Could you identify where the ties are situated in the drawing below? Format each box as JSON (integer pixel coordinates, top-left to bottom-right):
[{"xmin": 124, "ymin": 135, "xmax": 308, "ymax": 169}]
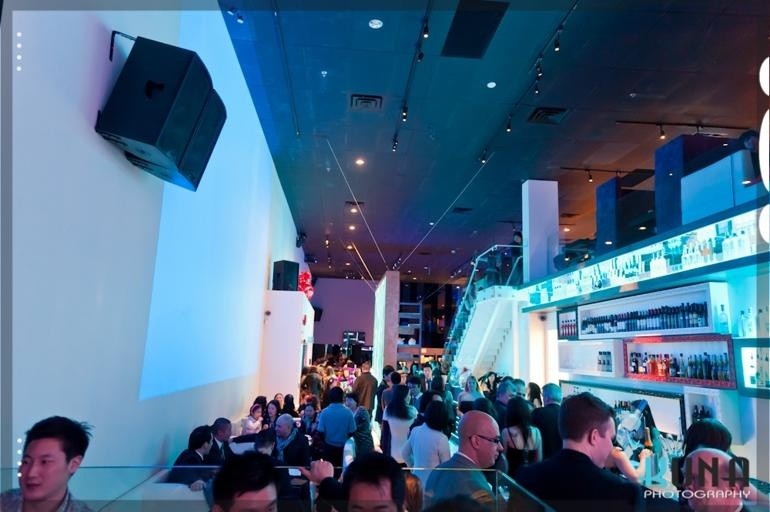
[{"xmin": 220, "ymin": 445, "xmax": 226, "ymax": 459}]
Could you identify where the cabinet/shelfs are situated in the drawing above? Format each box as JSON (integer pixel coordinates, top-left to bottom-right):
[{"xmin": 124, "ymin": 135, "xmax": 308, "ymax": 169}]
[{"xmin": 556, "ymin": 281, "xmax": 731, "ymax": 442}]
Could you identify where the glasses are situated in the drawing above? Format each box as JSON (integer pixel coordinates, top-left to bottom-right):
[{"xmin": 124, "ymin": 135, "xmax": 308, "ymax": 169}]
[{"xmin": 476, "ymin": 434, "xmax": 501, "ymax": 444}]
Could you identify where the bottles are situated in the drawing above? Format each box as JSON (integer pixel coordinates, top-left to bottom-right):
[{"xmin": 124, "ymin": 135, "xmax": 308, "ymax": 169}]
[
  {"xmin": 573, "ymin": 386, "xmax": 592, "ymax": 397},
  {"xmin": 692, "ymin": 404, "xmax": 710, "ymax": 423},
  {"xmin": 580, "ymin": 300, "xmax": 708, "ymax": 335},
  {"xmin": 614, "ymin": 399, "xmax": 631, "ymax": 424},
  {"xmin": 750, "ymin": 354, "xmax": 769, "ymax": 387},
  {"xmin": 629, "ymin": 352, "xmax": 730, "ymax": 382},
  {"xmin": 553, "ymin": 219, "xmax": 752, "ymax": 297},
  {"xmin": 560, "ymin": 318, "xmax": 576, "ymax": 336},
  {"xmin": 596, "ymin": 351, "xmax": 612, "ymax": 373},
  {"xmin": 644, "ymin": 427, "xmax": 654, "ymax": 454},
  {"xmin": 719, "ymin": 304, "xmax": 769, "ymax": 338}
]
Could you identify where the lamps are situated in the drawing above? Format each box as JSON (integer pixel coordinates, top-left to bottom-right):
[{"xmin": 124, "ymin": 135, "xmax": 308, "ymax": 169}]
[
  {"xmin": 528, "ymin": 1, "xmax": 581, "ymax": 74},
  {"xmin": 392, "ymin": 1, "xmax": 445, "ymax": 152},
  {"xmin": 659, "ymin": 125, "xmax": 665, "ymax": 140},
  {"xmin": 478, "ymin": 73, "xmax": 553, "ymax": 164},
  {"xmin": 588, "ymin": 170, "xmax": 593, "ymax": 183}
]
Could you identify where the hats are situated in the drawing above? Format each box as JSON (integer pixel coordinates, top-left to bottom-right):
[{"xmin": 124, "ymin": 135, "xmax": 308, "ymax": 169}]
[{"xmin": 425, "ymin": 401, "xmax": 448, "ymax": 425}]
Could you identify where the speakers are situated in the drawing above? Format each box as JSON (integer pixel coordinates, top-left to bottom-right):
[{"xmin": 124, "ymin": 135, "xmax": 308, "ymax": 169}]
[
  {"xmin": 273, "ymin": 259, "xmax": 300, "ymax": 291},
  {"xmin": 595, "ymin": 177, "xmax": 659, "ymax": 258},
  {"xmin": 652, "ymin": 133, "xmax": 744, "ymax": 233},
  {"xmin": 313, "ymin": 306, "xmax": 324, "ymax": 322},
  {"xmin": 94, "ymin": 32, "xmax": 215, "ymax": 189},
  {"xmin": 125, "ymin": 89, "xmax": 228, "ymax": 192}
]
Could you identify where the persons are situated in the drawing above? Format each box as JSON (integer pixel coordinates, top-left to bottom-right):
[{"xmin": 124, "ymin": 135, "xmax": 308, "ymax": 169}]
[
  {"xmin": 164, "ymin": 346, "xmax": 661, "ymax": 510},
  {"xmin": 0, "ymin": 416, "xmax": 92, "ymax": 512},
  {"xmin": 510, "ymin": 232, "xmax": 523, "ymax": 285},
  {"xmin": 680, "ymin": 447, "xmax": 751, "ymax": 512},
  {"xmin": 737, "ymin": 130, "xmax": 761, "ymax": 178},
  {"xmin": 672, "ymin": 418, "xmax": 770, "ymax": 512}
]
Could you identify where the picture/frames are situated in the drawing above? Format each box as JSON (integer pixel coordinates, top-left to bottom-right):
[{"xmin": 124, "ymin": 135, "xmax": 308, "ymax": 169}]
[{"xmin": 732, "ymin": 338, "xmax": 770, "ymax": 400}]
